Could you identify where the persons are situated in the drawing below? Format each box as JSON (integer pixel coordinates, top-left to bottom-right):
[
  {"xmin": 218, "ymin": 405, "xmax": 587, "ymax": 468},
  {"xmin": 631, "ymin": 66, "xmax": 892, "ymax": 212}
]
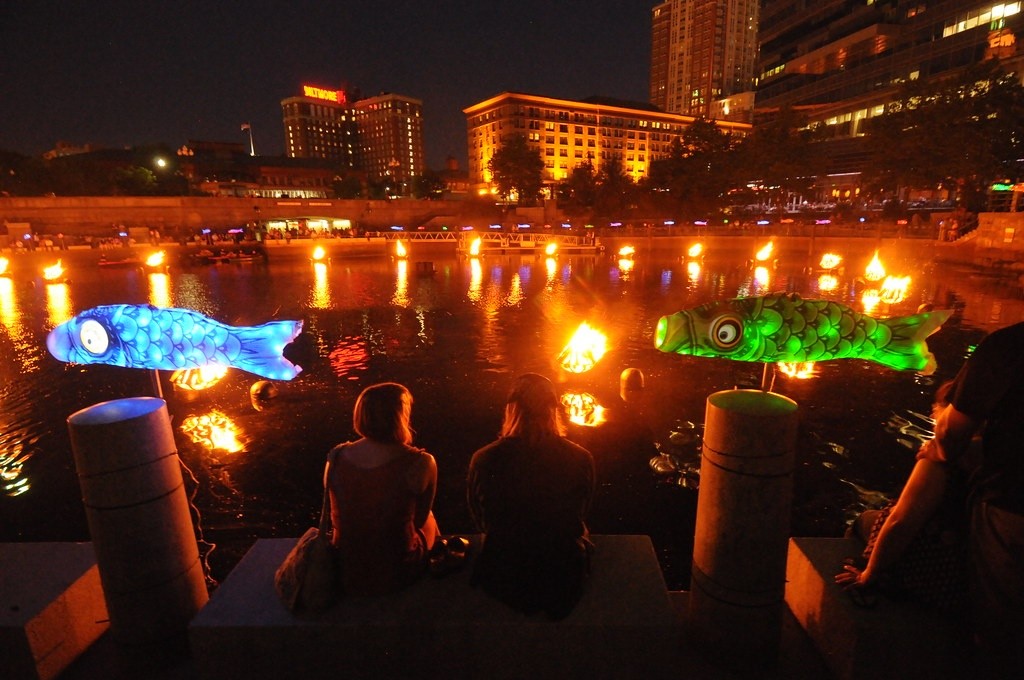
[
  {"xmin": 325, "ymin": 379, "xmax": 443, "ymax": 594},
  {"xmin": 231, "ymin": 224, "xmax": 371, "ymax": 243},
  {"xmin": 913, "ymin": 319, "xmax": 1023, "ymax": 671},
  {"xmin": 466, "ymin": 371, "xmax": 598, "ymax": 615},
  {"xmin": 836, "ymin": 377, "xmax": 988, "ymax": 614}
]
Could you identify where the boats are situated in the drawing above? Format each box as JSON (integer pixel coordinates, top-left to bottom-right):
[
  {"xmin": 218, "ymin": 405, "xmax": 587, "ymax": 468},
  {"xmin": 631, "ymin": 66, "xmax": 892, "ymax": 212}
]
[{"xmin": 190, "ymin": 226, "xmax": 266, "ymax": 263}]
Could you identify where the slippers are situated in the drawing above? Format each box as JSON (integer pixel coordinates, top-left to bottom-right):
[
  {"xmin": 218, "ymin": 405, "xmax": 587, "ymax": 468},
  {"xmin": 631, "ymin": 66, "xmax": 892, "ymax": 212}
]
[{"xmin": 430, "ymin": 535, "xmax": 470, "ymax": 578}]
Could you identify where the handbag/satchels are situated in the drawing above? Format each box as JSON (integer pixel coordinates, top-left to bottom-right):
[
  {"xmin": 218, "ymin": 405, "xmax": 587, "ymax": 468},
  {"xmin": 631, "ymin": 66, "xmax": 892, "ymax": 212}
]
[{"xmin": 274, "ymin": 441, "xmax": 351, "ymax": 618}]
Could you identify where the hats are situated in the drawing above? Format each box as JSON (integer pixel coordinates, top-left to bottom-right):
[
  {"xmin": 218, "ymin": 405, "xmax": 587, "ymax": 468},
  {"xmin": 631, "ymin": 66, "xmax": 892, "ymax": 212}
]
[{"xmin": 507, "ymin": 373, "xmax": 559, "ymax": 408}]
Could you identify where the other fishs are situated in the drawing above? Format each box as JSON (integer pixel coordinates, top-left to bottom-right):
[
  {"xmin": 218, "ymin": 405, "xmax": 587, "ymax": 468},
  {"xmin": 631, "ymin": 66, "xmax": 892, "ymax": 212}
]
[
  {"xmin": 45, "ymin": 301, "xmax": 305, "ymax": 384},
  {"xmin": 655, "ymin": 291, "xmax": 955, "ymax": 375}
]
